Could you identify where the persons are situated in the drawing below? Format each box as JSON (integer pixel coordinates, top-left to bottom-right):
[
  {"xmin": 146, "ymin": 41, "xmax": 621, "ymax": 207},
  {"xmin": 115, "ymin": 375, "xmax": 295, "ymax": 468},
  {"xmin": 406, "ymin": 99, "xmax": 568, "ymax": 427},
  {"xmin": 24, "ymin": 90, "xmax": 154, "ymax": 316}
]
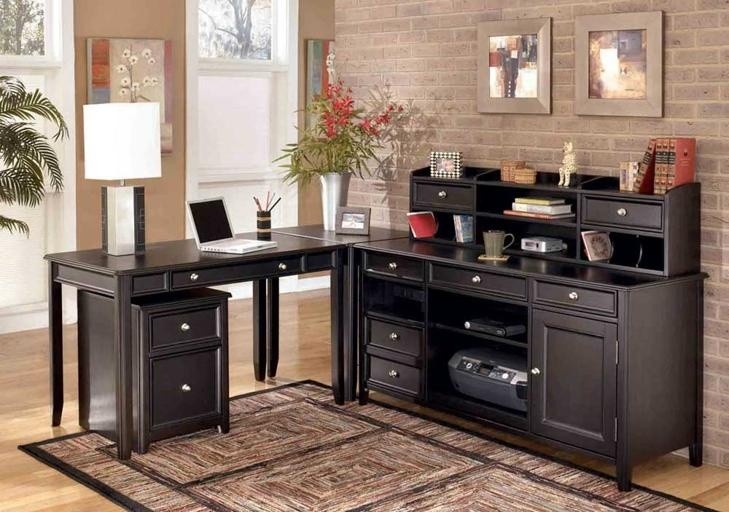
[{"xmin": 436, "ymin": 157, "xmax": 453, "ymax": 172}]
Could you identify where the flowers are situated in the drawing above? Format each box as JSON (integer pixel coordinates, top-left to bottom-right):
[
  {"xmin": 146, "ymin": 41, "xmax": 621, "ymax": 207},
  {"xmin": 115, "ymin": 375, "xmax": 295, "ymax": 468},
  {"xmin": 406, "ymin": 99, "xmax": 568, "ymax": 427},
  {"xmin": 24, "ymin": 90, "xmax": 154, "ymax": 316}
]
[{"xmin": 269, "ymin": 71, "xmax": 413, "ymax": 188}]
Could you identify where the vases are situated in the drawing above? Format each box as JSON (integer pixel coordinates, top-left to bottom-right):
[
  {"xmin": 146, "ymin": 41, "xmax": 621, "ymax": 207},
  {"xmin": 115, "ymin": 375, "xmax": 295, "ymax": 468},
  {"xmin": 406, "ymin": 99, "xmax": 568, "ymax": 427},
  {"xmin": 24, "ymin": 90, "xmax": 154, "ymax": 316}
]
[{"xmin": 319, "ymin": 170, "xmax": 352, "ymax": 232}]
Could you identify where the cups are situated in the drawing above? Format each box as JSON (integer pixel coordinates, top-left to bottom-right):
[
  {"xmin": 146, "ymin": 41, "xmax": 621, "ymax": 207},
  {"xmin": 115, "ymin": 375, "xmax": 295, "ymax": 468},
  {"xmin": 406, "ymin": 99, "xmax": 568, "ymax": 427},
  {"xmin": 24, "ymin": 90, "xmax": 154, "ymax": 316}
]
[
  {"xmin": 256, "ymin": 210, "xmax": 271, "ymax": 240},
  {"xmin": 482, "ymin": 230, "xmax": 515, "ymax": 258}
]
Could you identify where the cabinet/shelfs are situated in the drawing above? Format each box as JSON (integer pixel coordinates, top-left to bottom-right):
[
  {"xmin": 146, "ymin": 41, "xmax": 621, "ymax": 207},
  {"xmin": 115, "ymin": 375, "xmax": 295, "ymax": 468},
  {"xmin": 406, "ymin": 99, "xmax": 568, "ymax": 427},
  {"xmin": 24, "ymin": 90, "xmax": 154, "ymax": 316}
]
[
  {"xmin": 353, "ymin": 165, "xmax": 710, "ymax": 493},
  {"xmin": 77, "ymin": 286, "xmax": 232, "ymax": 455}
]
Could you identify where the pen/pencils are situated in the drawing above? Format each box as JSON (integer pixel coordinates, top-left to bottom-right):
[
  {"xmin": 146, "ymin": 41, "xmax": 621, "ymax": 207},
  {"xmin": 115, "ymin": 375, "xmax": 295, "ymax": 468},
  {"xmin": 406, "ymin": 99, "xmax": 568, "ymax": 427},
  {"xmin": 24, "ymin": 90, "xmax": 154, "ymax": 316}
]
[
  {"xmin": 264, "ymin": 193, "xmax": 276, "ymax": 212},
  {"xmin": 266, "ymin": 191, "xmax": 270, "ymax": 213},
  {"xmin": 268, "ymin": 197, "xmax": 281, "ymax": 212},
  {"xmin": 253, "ymin": 196, "xmax": 263, "ymax": 212}
]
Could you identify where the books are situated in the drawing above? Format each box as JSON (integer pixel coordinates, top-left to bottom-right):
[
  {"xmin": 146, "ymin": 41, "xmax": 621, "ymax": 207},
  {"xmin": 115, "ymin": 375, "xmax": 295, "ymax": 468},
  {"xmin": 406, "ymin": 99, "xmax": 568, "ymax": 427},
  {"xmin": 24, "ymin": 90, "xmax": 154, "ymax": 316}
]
[
  {"xmin": 620, "ymin": 137, "xmax": 697, "ymax": 195},
  {"xmin": 503, "ymin": 194, "xmax": 575, "ymax": 220}
]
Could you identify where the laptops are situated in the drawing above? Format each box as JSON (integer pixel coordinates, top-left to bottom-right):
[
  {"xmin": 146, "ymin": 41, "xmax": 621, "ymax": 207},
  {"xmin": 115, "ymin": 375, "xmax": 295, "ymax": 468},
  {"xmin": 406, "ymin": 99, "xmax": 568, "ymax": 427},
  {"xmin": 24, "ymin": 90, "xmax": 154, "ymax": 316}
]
[{"xmin": 182, "ymin": 195, "xmax": 279, "ymax": 255}]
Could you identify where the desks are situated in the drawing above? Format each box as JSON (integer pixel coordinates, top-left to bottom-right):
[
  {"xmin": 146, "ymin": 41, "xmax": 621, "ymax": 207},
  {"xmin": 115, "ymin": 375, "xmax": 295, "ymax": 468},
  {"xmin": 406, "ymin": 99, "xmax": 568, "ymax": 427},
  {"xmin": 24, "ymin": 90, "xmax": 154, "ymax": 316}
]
[
  {"xmin": 44, "ymin": 228, "xmax": 347, "ymax": 461},
  {"xmin": 267, "ymin": 223, "xmax": 410, "ymax": 402}
]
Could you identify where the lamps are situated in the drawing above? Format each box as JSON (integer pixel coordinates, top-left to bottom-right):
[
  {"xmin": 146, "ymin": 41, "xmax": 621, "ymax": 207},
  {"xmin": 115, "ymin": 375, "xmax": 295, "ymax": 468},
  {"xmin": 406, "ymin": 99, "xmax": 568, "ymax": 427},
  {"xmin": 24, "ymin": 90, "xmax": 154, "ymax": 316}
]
[{"xmin": 82, "ymin": 100, "xmax": 163, "ymax": 257}]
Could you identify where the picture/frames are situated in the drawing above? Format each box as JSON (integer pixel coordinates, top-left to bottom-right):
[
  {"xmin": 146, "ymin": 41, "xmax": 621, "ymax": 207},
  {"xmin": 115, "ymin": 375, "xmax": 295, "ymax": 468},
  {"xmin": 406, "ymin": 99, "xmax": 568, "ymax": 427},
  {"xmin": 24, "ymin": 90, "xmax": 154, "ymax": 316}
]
[
  {"xmin": 335, "ymin": 205, "xmax": 371, "ymax": 237},
  {"xmin": 429, "ymin": 150, "xmax": 464, "ymax": 178},
  {"xmin": 574, "ymin": 10, "xmax": 663, "ymax": 118},
  {"xmin": 476, "ymin": 16, "xmax": 552, "ymax": 115}
]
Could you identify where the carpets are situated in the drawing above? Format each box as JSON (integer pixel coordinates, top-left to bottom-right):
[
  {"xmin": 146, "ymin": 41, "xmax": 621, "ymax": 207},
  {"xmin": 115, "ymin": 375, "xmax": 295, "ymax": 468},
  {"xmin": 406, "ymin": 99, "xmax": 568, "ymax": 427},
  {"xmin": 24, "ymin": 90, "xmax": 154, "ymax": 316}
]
[{"xmin": 17, "ymin": 379, "xmax": 717, "ymax": 512}]
